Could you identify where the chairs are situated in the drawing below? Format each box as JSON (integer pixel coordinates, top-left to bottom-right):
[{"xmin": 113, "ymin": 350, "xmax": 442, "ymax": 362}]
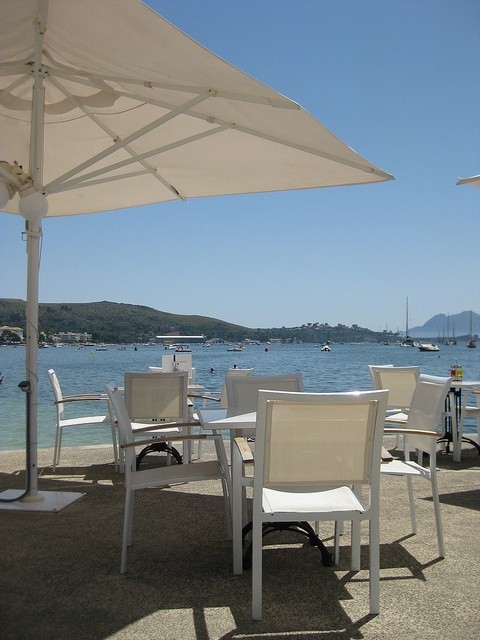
[
  {"xmin": 367, "ymin": 362, "xmax": 409, "ymax": 449},
  {"xmin": 225, "ymin": 374, "xmax": 324, "ymax": 542},
  {"xmin": 190, "ymin": 368, "xmax": 252, "ymax": 460},
  {"xmin": 231, "ymin": 390, "xmax": 389, "ymax": 620},
  {"xmin": 335, "ymin": 373, "xmax": 452, "ymax": 565},
  {"xmin": 370, "ymin": 366, "xmax": 424, "ymax": 467},
  {"xmin": 47, "ymin": 368, "xmax": 120, "ymax": 471},
  {"xmin": 106, "ymin": 381, "xmax": 247, "ymax": 573},
  {"xmin": 119, "ymin": 371, "xmax": 190, "ymax": 484}
]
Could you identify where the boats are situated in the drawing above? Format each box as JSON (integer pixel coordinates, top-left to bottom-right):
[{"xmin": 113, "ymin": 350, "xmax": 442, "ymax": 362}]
[
  {"xmin": 401, "ymin": 337, "xmax": 419, "ymax": 347},
  {"xmin": 417, "ymin": 344, "xmax": 439, "ymax": 351},
  {"xmin": 467, "ymin": 342, "xmax": 476, "ymax": 348},
  {"xmin": 228, "ymin": 344, "xmax": 245, "ymax": 351},
  {"xmin": 176, "ymin": 347, "xmax": 192, "ymax": 352}
]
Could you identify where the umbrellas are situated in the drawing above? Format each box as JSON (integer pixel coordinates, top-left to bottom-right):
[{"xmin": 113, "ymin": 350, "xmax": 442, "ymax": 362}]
[{"xmin": 0, "ymin": 2, "xmax": 394, "ymax": 512}]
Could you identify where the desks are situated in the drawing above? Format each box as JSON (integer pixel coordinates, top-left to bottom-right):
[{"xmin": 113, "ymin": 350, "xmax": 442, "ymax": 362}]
[
  {"xmin": 116, "ymin": 385, "xmax": 204, "ymax": 471},
  {"xmin": 437, "ymin": 381, "xmax": 480, "ymax": 462},
  {"xmin": 198, "ymin": 409, "xmax": 333, "ymax": 569}
]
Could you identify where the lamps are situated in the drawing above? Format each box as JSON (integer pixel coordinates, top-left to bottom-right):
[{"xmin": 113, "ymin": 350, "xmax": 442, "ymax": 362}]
[
  {"xmin": 18, "ymin": 182, "xmax": 48, "ymax": 220},
  {"xmin": 1, "ymin": 181, "xmax": 15, "ymax": 209}
]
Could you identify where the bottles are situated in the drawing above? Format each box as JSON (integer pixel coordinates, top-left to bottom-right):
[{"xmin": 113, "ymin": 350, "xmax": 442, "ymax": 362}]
[
  {"xmin": 450, "ymin": 358, "xmax": 457, "ymax": 381},
  {"xmin": 457, "ymin": 365, "xmax": 463, "ymax": 381}
]
